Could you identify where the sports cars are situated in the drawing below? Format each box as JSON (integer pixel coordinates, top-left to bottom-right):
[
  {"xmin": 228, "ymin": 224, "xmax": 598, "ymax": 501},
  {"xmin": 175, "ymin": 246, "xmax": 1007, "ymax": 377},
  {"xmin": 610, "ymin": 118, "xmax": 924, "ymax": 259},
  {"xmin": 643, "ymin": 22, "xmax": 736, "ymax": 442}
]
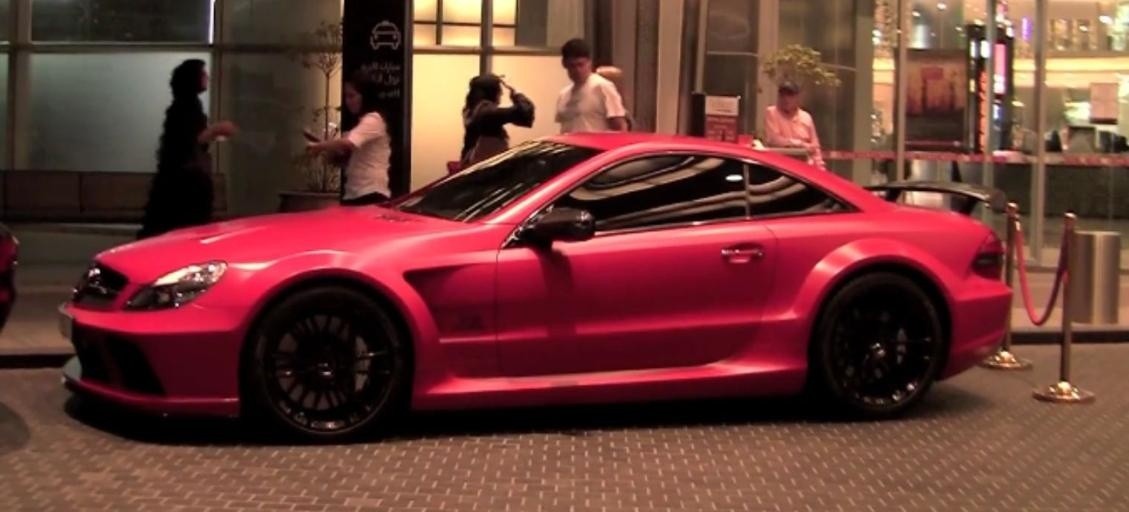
[{"xmin": 55, "ymin": 130, "xmax": 1010, "ymax": 444}]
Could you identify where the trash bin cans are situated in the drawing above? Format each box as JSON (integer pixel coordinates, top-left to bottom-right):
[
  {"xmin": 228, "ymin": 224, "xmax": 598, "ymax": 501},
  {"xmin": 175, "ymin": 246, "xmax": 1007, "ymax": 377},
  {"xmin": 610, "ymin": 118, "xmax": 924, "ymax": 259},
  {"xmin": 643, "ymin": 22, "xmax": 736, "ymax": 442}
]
[{"xmin": 1069, "ymin": 230, "xmax": 1122, "ymax": 322}]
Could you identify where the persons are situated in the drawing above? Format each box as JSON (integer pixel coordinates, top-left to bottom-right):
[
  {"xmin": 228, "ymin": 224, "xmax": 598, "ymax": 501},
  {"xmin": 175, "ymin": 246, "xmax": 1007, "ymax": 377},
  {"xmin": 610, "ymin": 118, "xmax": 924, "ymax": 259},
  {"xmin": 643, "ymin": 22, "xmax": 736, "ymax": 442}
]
[
  {"xmin": 136, "ymin": 58, "xmax": 242, "ymax": 243},
  {"xmin": 302, "ymin": 73, "xmax": 391, "ymax": 204},
  {"xmin": 462, "ymin": 75, "xmax": 535, "ymax": 172},
  {"xmin": 552, "ymin": 39, "xmax": 628, "ymax": 131},
  {"xmin": 763, "ymin": 79, "xmax": 826, "ymax": 170},
  {"xmin": 595, "ymin": 66, "xmax": 647, "ymax": 131}
]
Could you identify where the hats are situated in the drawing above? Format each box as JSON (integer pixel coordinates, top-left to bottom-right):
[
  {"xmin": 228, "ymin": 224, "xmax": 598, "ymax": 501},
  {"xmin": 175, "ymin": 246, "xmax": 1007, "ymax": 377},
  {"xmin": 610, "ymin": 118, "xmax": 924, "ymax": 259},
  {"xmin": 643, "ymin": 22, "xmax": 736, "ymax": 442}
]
[{"xmin": 778, "ymin": 78, "xmax": 800, "ymax": 94}]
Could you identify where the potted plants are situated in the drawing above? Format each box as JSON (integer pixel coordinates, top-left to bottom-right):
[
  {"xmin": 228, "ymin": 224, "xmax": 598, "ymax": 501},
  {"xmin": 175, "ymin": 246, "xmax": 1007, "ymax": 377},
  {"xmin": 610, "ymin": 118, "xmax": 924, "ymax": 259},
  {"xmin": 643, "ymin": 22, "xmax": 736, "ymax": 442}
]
[{"xmin": 271, "ymin": 16, "xmax": 348, "ymax": 211}]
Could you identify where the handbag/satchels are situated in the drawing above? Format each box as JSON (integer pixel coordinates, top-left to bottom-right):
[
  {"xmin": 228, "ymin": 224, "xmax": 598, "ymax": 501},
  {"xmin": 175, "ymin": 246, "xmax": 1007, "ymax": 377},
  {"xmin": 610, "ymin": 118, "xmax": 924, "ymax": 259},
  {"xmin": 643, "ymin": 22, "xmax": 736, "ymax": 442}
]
[{"xmin": 455, "ymin": 99, "xmax": 509, "ymax": 171}]
